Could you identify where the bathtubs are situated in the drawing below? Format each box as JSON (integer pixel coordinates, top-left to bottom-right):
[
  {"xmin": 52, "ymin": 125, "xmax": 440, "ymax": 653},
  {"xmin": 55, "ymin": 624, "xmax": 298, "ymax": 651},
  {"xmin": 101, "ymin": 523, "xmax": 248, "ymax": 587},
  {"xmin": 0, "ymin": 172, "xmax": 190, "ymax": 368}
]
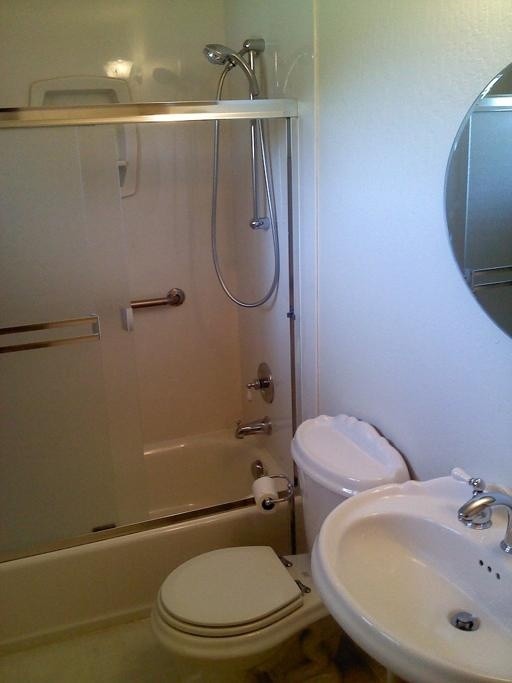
[{"xmin": 0, "ymin": 426, "xmax": 294, "ymax": 654}]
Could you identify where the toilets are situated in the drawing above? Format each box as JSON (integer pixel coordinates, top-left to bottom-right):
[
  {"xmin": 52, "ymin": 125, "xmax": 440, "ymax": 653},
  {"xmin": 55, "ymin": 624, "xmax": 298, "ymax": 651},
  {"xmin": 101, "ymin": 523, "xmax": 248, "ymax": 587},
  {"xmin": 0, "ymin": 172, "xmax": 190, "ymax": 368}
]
[{"xmin": 149, "ymin": 411, "xmax": 411, "ymax": 681}]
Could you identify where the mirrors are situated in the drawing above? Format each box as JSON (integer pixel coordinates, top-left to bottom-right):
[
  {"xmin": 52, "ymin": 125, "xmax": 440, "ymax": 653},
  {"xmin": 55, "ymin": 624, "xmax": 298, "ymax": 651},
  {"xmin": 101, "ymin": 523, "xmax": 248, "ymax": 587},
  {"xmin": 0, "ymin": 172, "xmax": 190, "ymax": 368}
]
[{"xmin": 443, "ymin": 63, "xmax": 512, "ymax": 341}]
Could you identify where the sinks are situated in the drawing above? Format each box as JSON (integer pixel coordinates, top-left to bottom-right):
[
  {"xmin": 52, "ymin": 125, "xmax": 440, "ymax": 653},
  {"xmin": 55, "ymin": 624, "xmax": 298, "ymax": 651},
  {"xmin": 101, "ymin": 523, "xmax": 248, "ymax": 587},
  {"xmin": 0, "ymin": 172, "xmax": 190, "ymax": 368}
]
[{"xmin": 309, "ymin": 466, "xmax": 512, "ymax": 682}]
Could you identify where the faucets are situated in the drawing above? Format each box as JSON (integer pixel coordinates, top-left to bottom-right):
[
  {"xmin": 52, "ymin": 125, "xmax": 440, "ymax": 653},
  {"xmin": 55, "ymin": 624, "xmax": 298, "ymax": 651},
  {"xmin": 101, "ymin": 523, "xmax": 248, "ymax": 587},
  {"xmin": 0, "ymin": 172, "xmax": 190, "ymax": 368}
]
[
  {"xmin": 458, "ymin": 491, "xmax": 512, "ymax": 554},
  {"xmin": 236, "ymin": 416, "xmax": 273, "ymax": 440}
]
